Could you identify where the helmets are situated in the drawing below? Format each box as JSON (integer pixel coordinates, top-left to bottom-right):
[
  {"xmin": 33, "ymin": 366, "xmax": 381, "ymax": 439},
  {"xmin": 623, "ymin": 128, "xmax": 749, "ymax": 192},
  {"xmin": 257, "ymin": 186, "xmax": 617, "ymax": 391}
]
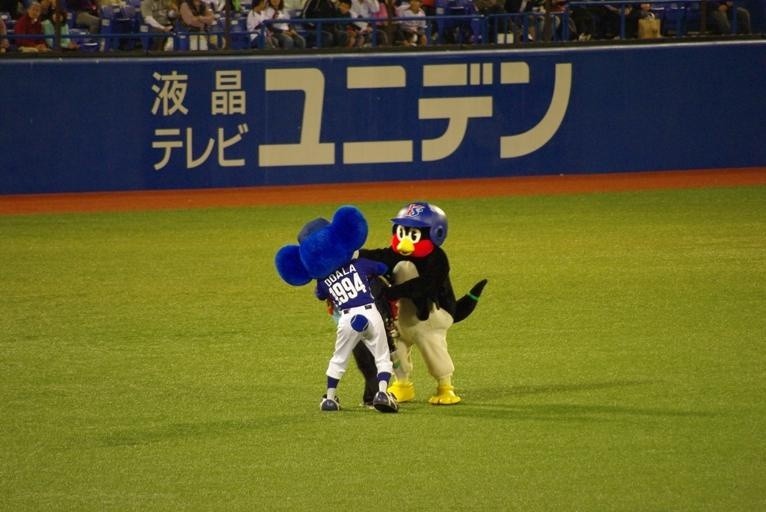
[{"xmin": 392, "ymin": 202, "xmax": 447, "ymax": 241}]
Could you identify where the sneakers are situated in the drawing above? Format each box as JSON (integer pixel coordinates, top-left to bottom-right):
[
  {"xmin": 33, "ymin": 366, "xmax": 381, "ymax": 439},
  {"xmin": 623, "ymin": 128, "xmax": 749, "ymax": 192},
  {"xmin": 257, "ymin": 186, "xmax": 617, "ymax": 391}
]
[
  {"xmin": 376, "ymin": 392, "xmax": 398, "ymax": 412},
  {"xmin": 321, "ymin": 394, "xmax": 338, "ymax": 412}
]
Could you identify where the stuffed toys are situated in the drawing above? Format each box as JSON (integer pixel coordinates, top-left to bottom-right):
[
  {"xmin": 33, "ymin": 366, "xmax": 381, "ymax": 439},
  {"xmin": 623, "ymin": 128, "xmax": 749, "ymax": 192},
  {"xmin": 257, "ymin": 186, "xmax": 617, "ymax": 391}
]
[
  {"xmin": 273, "ymin": 203, "xmax": 402, "ymax": 415},
  {"xmin": 377, "ymin": 202, "xmax": 489, "ymax": 403}
]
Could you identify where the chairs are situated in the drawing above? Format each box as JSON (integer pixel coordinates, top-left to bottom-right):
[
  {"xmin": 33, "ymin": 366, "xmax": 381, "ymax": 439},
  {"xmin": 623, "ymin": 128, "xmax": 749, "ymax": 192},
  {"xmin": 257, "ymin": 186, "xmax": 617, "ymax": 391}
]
[{"xmin": 0, "ymin": 0, "xmax": 752, "ymax": 52}]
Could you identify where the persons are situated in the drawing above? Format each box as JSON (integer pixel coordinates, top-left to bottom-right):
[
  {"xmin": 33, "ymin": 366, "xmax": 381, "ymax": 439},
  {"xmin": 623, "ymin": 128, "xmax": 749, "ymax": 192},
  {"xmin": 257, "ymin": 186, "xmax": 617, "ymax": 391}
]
[
  {"xmin": 2, "ymin": 0, "xmax": 765, "ymax": 61},
  {"xmin": 330, "ymin": 272, "xmax": 402, "ymax": 410}
]
